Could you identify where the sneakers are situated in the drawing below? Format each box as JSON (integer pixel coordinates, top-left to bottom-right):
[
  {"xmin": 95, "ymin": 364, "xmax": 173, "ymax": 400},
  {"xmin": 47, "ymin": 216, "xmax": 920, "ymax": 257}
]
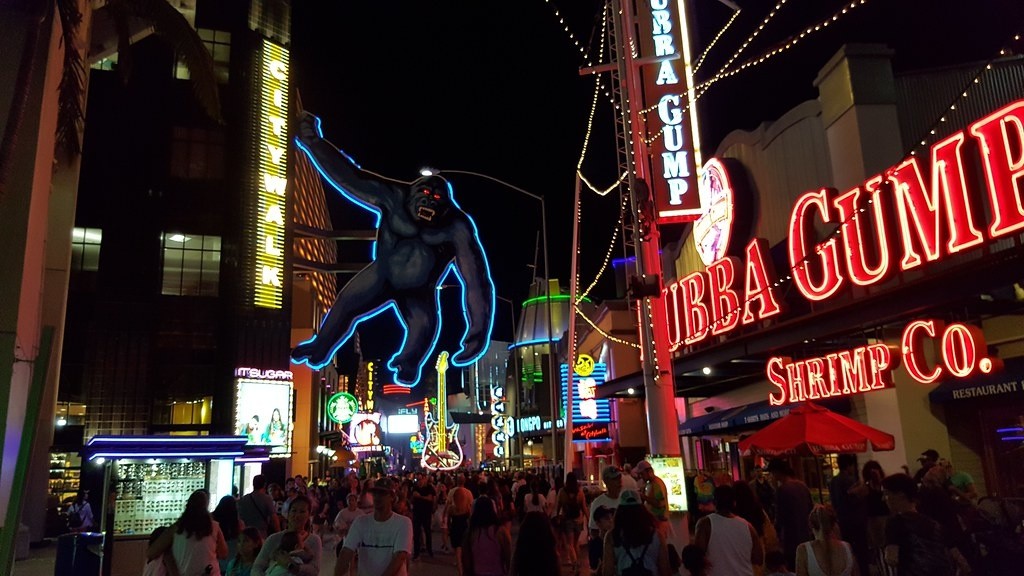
[
  {"xmin": 428, "ymin": 549, "xmax": 434, "ymax": 556},
  {"xmin": 412, "ymin": 555, "xmax": 421, "ymax": 561}
]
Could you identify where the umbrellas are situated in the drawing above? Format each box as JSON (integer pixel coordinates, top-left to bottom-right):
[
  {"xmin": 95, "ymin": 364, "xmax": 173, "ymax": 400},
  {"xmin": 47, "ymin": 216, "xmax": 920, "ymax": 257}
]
[{"xmin": 738, "ymin": 397, "xmax": 896, "ymax": 473}]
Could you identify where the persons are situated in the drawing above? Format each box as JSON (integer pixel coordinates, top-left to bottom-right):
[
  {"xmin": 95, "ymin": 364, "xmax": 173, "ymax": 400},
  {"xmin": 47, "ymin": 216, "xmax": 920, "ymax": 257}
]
[
  {"xmin": 47, "ymin": 488, "xmax": 94, "ymax": 536},
  {"xmin": 143, "ymin": 449, "xmax": 986, "ymax": 576}
]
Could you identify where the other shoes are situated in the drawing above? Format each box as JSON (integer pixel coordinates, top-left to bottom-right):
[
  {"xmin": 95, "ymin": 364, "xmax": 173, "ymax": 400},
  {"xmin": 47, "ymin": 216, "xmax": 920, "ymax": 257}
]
[{"xmin": 441, "ymin": 545, "xmax": 449, "ymax": 554}]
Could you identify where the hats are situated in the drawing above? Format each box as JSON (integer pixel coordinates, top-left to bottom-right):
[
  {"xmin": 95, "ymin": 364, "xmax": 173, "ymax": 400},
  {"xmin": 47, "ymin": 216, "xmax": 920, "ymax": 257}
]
[
  {"xmin": 620, "ymin": 489, "xmax": 641, "ymax": 504},
  {"xmin": 78, "ymin": 487, "xmax": 88, "ymax": 493},
  {"xmin": 631, "ymin": 461, "xmax": 651, "ymax": 473},
  {"xmin": 922, "ymin": 449, "xmax": 939, "ymax": 456},
  {"xmin": 768, "ymin": 457, "xmax": 790, "ymax": 472},
  {"xmin": 594, "ymin": 504, "xmax": 616, "ymax": 522},
  {"xmin": 368, "ymin": 478, "xmax": 395, "ymax": 493},
  {"xmin": 603, "ymin": 466, "xmax": 622, "ymax": 479},
  {"xmin": 188, "ymin": 490, "xmax": 208, "ymax": 508}
]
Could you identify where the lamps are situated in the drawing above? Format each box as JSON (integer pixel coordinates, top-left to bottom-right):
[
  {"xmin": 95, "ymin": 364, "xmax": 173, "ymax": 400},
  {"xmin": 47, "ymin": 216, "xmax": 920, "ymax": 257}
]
[{"xmin": 1013, "ymin": 283, "xmax": 1024, "ymax": 300}]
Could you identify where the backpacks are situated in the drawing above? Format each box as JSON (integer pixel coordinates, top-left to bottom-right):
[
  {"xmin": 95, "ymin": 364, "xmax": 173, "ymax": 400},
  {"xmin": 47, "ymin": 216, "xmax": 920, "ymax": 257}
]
[
  {"xmin": 562, "ymin": 488, "xmax": 580, "ymax": 521},
  {"xmin": 613, "ymin": 545, "xmax": 654, "ymax": 576},
  {"xmin": 514, "ymin": 485, "xmax": 531, "ymax": 507},
  {"xmin": 67, "ymin": 501, "xmax": 88, "ymax": 527}
]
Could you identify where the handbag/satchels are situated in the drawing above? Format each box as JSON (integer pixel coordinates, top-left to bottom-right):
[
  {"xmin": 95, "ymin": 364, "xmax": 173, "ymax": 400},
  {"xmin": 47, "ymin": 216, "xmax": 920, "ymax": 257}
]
[{"xmin": 268, "ymin": 524, "xmax": 275, "ymax": 534}]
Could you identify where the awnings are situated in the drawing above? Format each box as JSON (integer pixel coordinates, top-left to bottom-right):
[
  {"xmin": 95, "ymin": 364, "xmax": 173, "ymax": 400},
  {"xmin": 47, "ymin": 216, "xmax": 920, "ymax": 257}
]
[
  {"xmin": 677, "ymin": 401, "xmax": 791, "ymax": 437},
  {"xmin": 928, "ymin": 353, "xmax": 1024, "ymax": 410}
]
